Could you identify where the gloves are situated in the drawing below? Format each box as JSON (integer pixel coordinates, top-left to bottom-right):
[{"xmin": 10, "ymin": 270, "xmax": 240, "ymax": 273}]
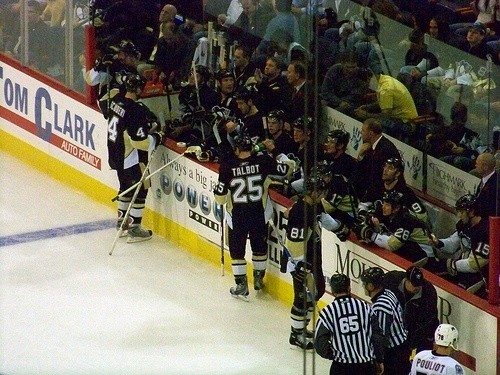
[
  {"xmin": 291, "ymin": 260, "xmax": 312, "ymax": 284},
  {"xmin": 434, "ymin": 258, "xmax": 458, "ymax": 277},
  {"xmin": 355, "ymin": 224, "xmax": 372, "ymax": 244},
  {"xmin": 332, "ymin": 219, "xmax": 350, "ymax": 242},
  {"xmin": 154, "ymin": 131, "xmax": 166, "ymax": 145}
]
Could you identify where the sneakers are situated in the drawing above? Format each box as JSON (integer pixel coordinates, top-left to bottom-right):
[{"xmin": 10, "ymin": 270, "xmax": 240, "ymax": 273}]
[
  {"xmin": 289, "ymin": 332, "xmax": 315, "ymax": 353},
  {"xmin": 127, "ymin": 224, "xmax": 152, "ymax": 244},
  {"xmin": 230, "ymin": 275, "xmax": 249, "ymax": 302},
  {"xmin": 254, "ymin": 270, "xmax": 266, "ymax": 294},
  {"xmin": 306, "ymin": 328, "xmax": 316, "ymax": 341},
  {"xmin": 117, "ymin": 221, "xmax": 129, "ymax": 238}
]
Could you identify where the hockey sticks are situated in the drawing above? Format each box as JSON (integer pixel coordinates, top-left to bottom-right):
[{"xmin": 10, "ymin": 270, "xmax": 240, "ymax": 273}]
[
  {"xmin": 267, "ymin": 220, "xmax": 321, "ymax": 313},
  {"xmin": 415, "ymin": 213, "xmax": 442, "ymax": 262},
  {"xmin": 465, "ymin": 217, "xmax": 491, "ymax": 294},
  {"xmin": 192, "ymin": 59, "xmax": 206, "ymax": 141},
  {"xmin": 220, "ymin": 203, "xmax": 224, "ymax": 278},
  {"xmin": 111, "ymin": 146, "xmax": 194, "ymax": 203},
  {"xmin": 333, "ymin": 173, "xmax": 362, "ymax": 223},
  {"xmin": 109, "ymin": 152, "xmax": 152, "ymax": 253}
]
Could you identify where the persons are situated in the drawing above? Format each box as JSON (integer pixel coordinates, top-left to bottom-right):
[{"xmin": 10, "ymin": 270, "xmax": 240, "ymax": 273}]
[
  {"xmin": 314, "ymin": 273, "xmax": 384, "ymax": 375},
  {"xmin": 1, "ymin": 0, "xmax": 500, "ymax": 290},
  {"xmin": 361, "ymin": 267, "xmax": 411, "ymax": 375},
  {"xmin": 213, "ymin": 133, "xmax": 295, "ymax": 302},
  {"xmin": 383, "ymin": 265, "xmax": 439, "ymax": 351},
  {"xmin": 288, "ymin": 175, "xmax": 328, "ymax": 354},
  {"xmin": 409, "ymin": 323, "xmax": 464, "ymax": 375}
]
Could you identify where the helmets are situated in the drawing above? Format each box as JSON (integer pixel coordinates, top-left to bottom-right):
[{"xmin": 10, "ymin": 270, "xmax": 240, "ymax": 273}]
[
  {"xmin": 361, "ymin": 267, "xmax": 385, "ymax": 283},
  {"xmin": 119, "ymin": 41, "xmax": 136, "ymax": 57},
  {"xmin": 267, "ymin": 109, "xmax": 287, "ymax": 123},
  {"xmin": 381, "ymin": 190, "xmax": 404, "ymax": 204},
  {"xmin": 317, "ymin": 160, "xmax": 335, "ymax": 176},
  {"xmin": 192, "ymin": 63, "xmax": 260, "ymax": 100},
  {"xmin": 384, "ymin": 157, "xmax": 405, "ymax": 172},
  {"xmin": 125, "ymin": 73, "xmax": 147, "ymax": 93},
  {"xmin": 306, "ymin": 176, "xmax": 328, "ymax": 191},
  {"xmin": 327, "ymin": 129, "xmax": 350, "ymax": 144},
  {"xmin": 456, "ymin": 194, "xmax": 479, "ymax": 211},
  {"xmin": 293, "ymin": 116, "xmax": 315, "ymax": 130},
  {"xmin": 331, "ymin": 274, "xmax": 349, "ymax": 287},
  {"xmin": 434, "ymin": 324, "xmax": 459, "ymax": 352},
  {"xmin": 234, "ymin": 134, "xmax": 252, "ymax": 152}
]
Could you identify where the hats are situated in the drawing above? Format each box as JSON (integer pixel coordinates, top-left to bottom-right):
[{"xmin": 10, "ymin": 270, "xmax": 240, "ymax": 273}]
[
  {"xmin": 406, "ymin": 266, "xmax": 425, "ymax": 286},
  {"xmin": 469, "ymin": 21, "xmax": 486, "ymax": 34}
]
